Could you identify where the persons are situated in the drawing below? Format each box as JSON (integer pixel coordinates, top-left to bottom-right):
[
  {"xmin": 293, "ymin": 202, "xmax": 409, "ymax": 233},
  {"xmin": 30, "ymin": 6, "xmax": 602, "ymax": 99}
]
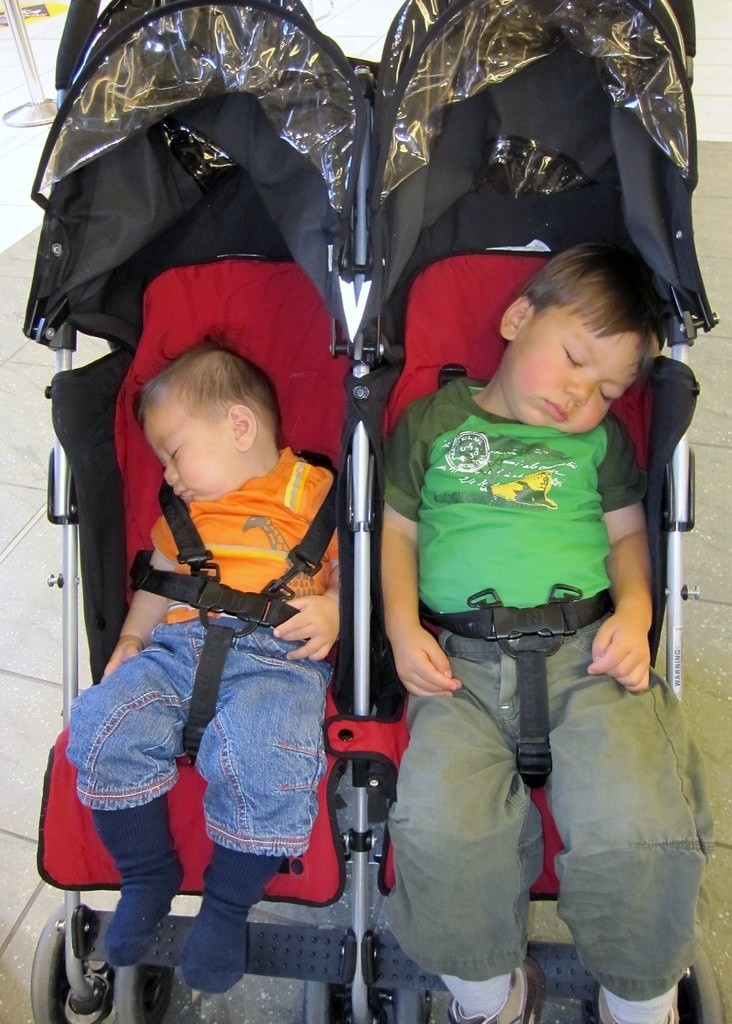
[
  {"xmin": 65, "ymin": 343, "xmax": 341, "ymax": 990},
  {"xmin": 381, "ymin": 240, "xmax": 713, "ymax": 1024}
]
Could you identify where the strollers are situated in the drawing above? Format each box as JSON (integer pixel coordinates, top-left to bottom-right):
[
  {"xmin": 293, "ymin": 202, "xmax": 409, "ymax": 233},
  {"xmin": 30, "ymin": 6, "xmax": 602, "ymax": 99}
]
[{"xmin": 31, "ymin": 0, "xmax": 706, "ymax": 1024}]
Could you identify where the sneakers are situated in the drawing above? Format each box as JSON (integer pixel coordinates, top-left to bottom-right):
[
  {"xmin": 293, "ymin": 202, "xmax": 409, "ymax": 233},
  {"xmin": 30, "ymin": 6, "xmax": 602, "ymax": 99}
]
[
  {"xmin": 449, "ymin": 956, "xmax": 546, "ymax": 1024},
  {"xmin": 598, "ymin": 983, "xmax": 680, "ymax": 1024}
]
